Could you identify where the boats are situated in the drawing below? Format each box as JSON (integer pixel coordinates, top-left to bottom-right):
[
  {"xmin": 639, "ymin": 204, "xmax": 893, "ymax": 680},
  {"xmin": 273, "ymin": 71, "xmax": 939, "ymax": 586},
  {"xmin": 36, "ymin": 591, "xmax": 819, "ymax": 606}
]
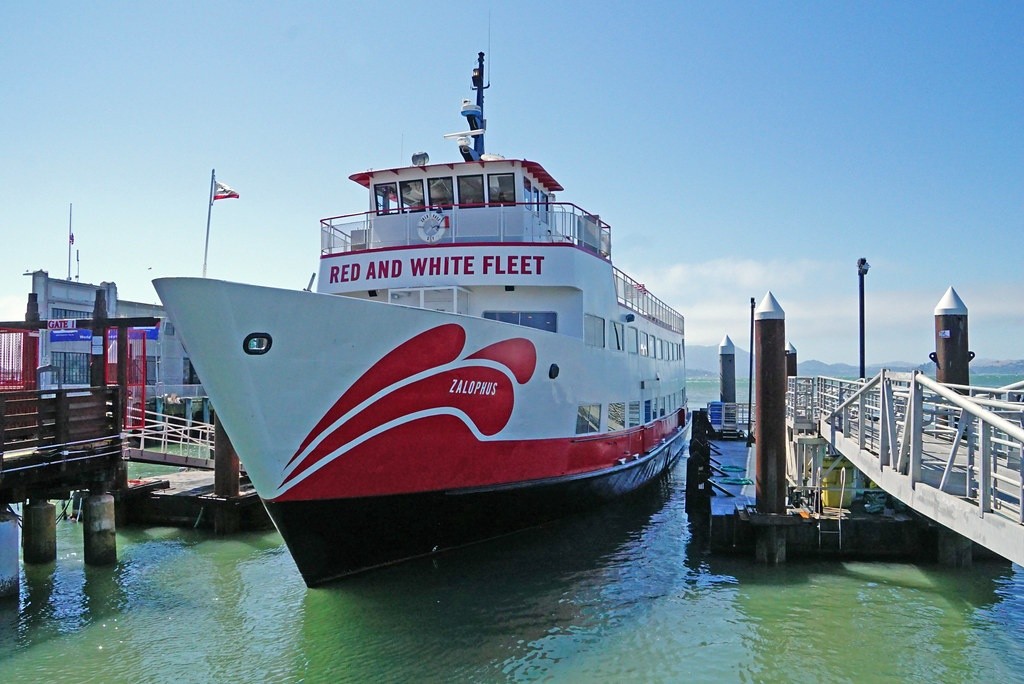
[{"xmin": 151, "ymin": 51, "xmax": 693, "ymax": 589}]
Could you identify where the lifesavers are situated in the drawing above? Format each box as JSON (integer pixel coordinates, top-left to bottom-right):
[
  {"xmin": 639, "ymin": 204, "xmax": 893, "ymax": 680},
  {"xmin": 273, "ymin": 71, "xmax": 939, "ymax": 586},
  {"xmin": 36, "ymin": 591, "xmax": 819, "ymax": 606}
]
[
  {"xmin": 416, "ymin": 212, "xmax": 445, "ymax": 243},
  {"xmin": 601, "ymin": 237, "xmax": 609, "ymax": 257}
]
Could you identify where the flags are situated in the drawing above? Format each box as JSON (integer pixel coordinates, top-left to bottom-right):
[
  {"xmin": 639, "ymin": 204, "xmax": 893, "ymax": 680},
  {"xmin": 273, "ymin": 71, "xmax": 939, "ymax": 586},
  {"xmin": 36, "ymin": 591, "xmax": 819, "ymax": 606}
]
[{"xmin": 211, "ymin": 176, "xmax": 239, "ymax": 203}]
